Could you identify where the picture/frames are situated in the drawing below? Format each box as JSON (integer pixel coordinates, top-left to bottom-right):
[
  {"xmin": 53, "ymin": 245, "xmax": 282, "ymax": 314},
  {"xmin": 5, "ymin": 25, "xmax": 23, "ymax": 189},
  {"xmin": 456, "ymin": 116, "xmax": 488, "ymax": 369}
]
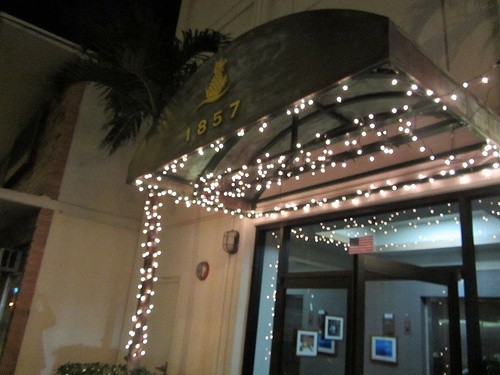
[
  {"xmin": 314, "ymin": 329, "xmax": 337, "ymax": 356},
  {"xmin": 294, "ymin": 327, "xmax": 319, "ymax": 356},
  {"xmin": 323, "ymin": 314, "xmax": 344, "ymax": 340},
  {"xmin": 369, "ymin": 334, "xmax": 399, "ymax": 364}
]
